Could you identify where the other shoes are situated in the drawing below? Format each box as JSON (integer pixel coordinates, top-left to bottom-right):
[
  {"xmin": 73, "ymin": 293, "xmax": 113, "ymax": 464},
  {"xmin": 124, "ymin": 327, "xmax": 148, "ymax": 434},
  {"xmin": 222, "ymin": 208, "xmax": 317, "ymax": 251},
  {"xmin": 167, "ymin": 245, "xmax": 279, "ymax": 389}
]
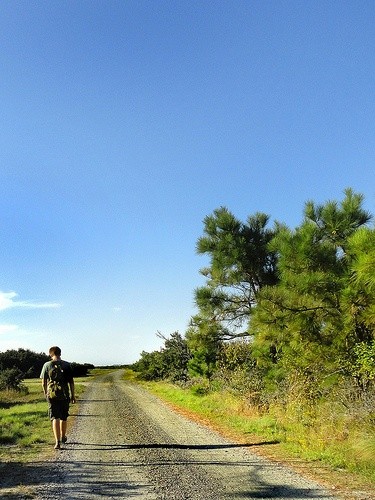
[
  {"xmin": 54, "ymin": 442, "xmax": 60, "ymax": 449},
  {"xmin": 62, "ymin": 436, "xmax": 67, "ymax": 444}
]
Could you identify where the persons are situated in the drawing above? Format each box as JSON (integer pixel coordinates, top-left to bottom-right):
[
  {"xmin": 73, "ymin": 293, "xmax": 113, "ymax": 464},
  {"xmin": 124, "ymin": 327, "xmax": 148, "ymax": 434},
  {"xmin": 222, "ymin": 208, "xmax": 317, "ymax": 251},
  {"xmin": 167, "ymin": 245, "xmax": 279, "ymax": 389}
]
[{"xmin": 40, "ymin": 346, "xmax": 75, "ymax": 449}]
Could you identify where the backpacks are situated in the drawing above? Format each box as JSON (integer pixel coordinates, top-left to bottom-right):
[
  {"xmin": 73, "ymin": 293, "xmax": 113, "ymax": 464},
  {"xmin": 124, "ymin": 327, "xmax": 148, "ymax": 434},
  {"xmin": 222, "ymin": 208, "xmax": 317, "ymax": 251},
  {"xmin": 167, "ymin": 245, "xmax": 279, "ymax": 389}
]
[{"xmin": 48, "ymin": 361, "xmax": 69, "ymax": 401}]
